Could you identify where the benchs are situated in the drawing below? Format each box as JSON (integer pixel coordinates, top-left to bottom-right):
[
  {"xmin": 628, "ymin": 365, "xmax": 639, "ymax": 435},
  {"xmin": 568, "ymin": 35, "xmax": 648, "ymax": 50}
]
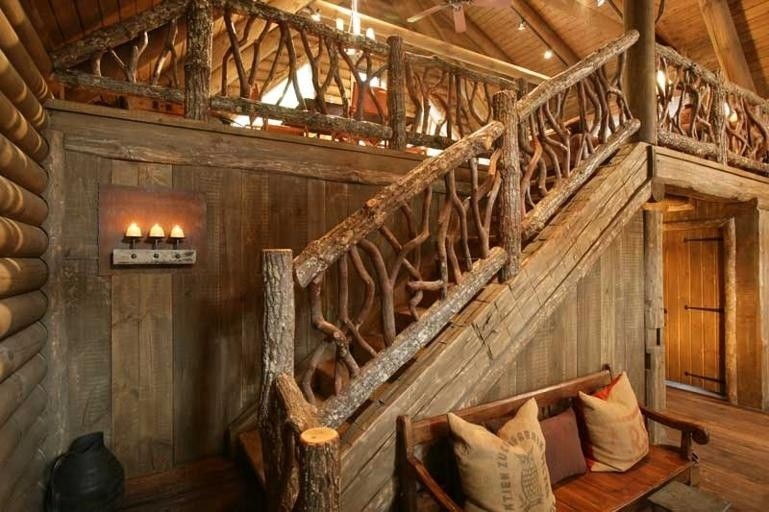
[{"xmin": 396, "ymin": 362, "xmax": 711, "ymax": 512}]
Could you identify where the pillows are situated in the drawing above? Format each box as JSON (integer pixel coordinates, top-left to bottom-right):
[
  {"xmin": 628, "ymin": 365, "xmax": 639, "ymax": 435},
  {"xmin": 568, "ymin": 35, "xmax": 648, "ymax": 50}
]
[{"xmin": 447, "ymin": 371, "xmax": 651, "ymax": 512}]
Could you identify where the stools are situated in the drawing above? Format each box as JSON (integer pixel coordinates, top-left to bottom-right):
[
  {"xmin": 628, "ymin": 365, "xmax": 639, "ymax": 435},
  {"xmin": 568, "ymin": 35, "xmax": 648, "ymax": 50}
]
[{"xmin": 647, "ymin": 479, "xmax": 733, "ymax": 512}]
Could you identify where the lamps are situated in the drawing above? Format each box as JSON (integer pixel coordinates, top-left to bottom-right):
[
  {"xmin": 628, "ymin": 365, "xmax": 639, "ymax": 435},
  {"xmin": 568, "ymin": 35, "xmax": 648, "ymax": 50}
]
[
  {"xmin": 113, "ymin": 221, "xmax": 198, "ymax": 266},
  {"xmin": 311, "ymin": 2, "xmax": 376, "ymax": 55},
  {"xmin": 517, "ymin": 18, "xmax": 525, "ymax": 31},
  {"xmin": 596, "ymin": 0, "xmax": 604, "ymax": 7}
]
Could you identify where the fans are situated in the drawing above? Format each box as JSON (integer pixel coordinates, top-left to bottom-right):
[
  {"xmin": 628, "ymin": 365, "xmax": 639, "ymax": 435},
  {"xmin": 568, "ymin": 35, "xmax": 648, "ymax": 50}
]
[{"xmin": 406, "ymin": 0, "xmax": 513, "ymax": 34}]
